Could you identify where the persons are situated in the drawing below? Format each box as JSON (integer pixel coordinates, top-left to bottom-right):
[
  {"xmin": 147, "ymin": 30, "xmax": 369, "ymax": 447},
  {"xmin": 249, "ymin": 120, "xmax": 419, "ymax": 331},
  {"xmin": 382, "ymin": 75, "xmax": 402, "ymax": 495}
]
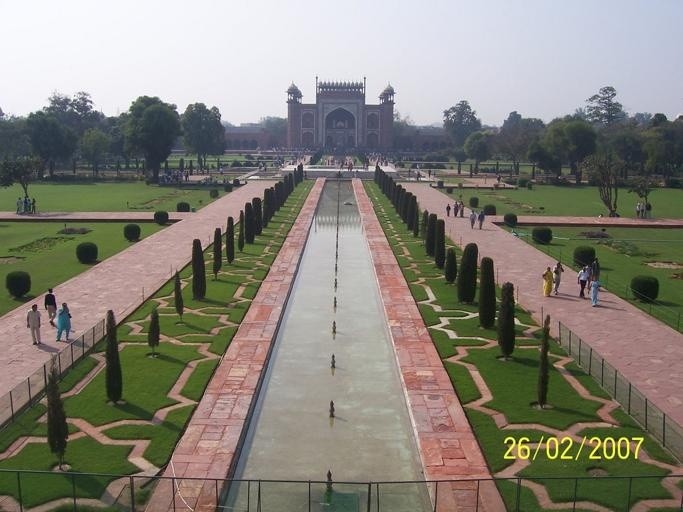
[
  {"xmin": 635, "ymin": 200, "xmax": 653, "ymax": 219},
  {"xmin": 589, "ymin": 276, "xmax": 600, "ymax": 307},
  {"xmin": 477, "ymin": 210, "xmax": 484, "ymax": 229},
  {"xmin": 584, "ymin": 257, "xmax": 600, "ymax": 294},
  {"xmin": 445, "ymin": 204, "xmax": 450, "ymax": 217},
  {"xmin": 469, "ymin": 210, "xmax": 476, "ymax": 230},
  {"xmin": 183, "ymin": 170, "xmax": 190, "ymax": 182},
  {"xmin": 458, "ymin": 201, "xmax": 464, "ymax": 217},
  {"xmin": 26, "ymin": 303, "xmax": 41, "ymax": 345},
  {"xmin": 496, "ymin": 174, "xmax": 501, "ymax": 183},
  {"xmin": 542, "ymin": 266, "xmax": 553, "ymax": 297},
  {"xmin": 22, "ymin": 195, "xmax": 31, "ymax": 215},
  {"xmin": 577, "ymin": 267, "xmax": 589, "ymax": 298},
  {"xmin": 54, "ymin": 302, "xmax": 72, "ymax": 342},
  {"xmin": 29, "ymin": 198, "xmax": 35, "ymax": 214},
  {"xmin": 14, "ymin": 197, "xmax": 22, "ymax": 214},
  {"xmin": 452, "ymin": 201, "xmax": 458, "ymax": 217},
  {"xmin": 43, "ymin": 287, "xmax": 56, "ymax": 325},
  {"xmin": 552, "ymin": 263, "xmax": 564, "ymax": 295}
]
